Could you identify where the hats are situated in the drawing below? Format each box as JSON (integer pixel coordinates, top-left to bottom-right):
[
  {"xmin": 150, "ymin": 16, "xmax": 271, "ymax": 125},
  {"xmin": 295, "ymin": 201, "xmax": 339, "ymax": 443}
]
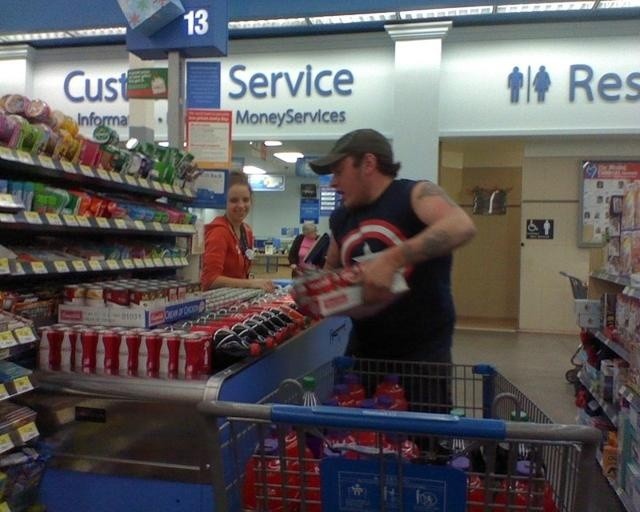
[
  {"xmin": 309, "ymin": 128, "xmax": 394, "ymax": 176},
  {"xmin": 302, "ymin": 224, "xmax": 318, "ymax": 235}
]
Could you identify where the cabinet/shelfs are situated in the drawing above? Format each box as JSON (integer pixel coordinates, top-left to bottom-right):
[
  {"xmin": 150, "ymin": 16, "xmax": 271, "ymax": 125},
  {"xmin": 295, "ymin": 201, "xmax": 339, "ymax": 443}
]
[
  {"xmin": 576, "ymin": 269, "xmax": 639, "ymax": 511},
  {"xmin": 0, "ymin": 144, "xmax": 196, "ymax": 510}
]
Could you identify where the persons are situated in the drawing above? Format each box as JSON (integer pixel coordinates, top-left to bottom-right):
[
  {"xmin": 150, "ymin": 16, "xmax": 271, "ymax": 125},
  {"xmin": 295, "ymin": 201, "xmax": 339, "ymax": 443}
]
[
  {"xmin": 201, "ymin": 170, "xmax": 275, "ymax": 293},
  {"xmin": 288, "ymin": 223, "xmax": 328, "ymax": 280},
  {"xmin": 310, "ymin": 129, "xmax": 476, "ymax": 414}
]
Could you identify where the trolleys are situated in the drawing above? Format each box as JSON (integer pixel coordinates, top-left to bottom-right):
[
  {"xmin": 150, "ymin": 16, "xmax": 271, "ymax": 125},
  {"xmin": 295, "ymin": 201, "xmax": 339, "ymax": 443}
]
[
  {"xmin": 196, "ymin": 356, "xmax": 602, "ymax": 511},
  {"xmin": 559, "ymin": 271, "xmax": 587, "ymax": 383}
]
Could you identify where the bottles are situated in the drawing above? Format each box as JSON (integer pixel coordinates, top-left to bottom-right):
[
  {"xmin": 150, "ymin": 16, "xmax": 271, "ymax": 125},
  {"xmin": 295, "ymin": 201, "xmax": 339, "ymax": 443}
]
[
  {"xmin": 183, "ymin": 264, "xmax": 348, "ymax": 367},
  {"xmin": 242, "ymin": 374, "xmax": 552, "ymax": 511}
]
